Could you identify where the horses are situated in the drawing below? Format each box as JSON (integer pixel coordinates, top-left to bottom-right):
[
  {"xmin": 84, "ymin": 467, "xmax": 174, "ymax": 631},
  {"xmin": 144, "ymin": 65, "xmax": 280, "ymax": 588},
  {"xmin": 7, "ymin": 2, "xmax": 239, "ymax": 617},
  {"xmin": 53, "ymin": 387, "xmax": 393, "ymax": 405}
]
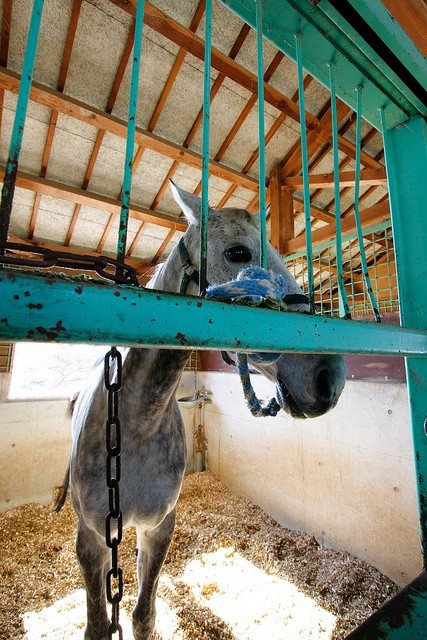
[{"xmin": 52, "ymin": 176, "xmax": 347, "ymax": 640}]
